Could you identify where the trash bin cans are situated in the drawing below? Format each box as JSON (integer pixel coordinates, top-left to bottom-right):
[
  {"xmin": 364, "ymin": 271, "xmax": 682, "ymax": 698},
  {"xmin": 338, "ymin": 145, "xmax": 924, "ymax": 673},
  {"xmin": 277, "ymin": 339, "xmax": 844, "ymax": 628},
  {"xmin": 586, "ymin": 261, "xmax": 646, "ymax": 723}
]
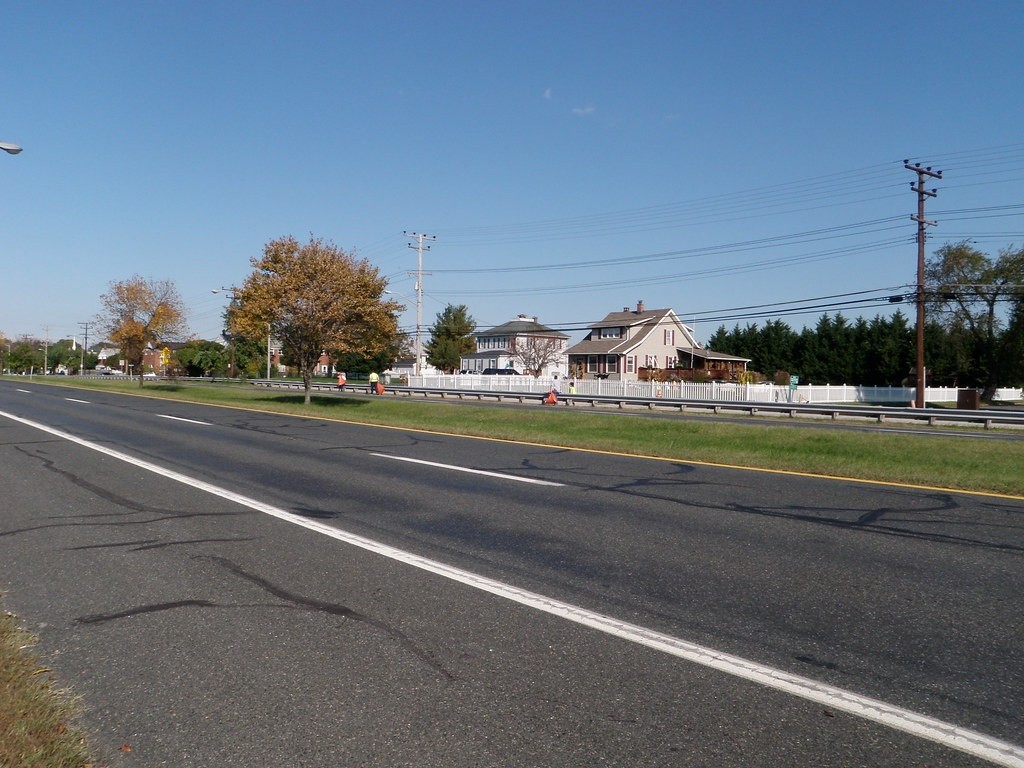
[{"xmin": 380, "ymin": 374, "xmax": 391, "ymax": 384}]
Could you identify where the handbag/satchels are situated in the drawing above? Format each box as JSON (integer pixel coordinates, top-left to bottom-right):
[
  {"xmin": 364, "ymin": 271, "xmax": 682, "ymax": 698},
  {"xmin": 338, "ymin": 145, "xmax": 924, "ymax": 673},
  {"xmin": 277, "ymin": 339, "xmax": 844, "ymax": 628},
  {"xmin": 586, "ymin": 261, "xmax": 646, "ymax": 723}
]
[
  {"xmin": 376, "ymin": 382, "xmax": 384, "ymax": 394},
  {"xmin": 544, "ymin": 392, "xmax": 556, "ymax": 404},
  {"xmin": 337, "ymin": 375, "xmax": 345, "ymax": 386}
]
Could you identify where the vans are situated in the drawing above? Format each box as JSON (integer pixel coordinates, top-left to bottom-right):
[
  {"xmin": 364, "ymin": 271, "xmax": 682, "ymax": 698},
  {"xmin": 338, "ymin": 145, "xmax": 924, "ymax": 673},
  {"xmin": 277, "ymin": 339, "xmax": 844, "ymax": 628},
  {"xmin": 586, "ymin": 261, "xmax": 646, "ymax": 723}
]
[
  {"xmin": 483, "ymin": 369, "xmax": 522, "ymax": 377},
  {"xmin": 458, "ymin": 369, "xmax": 478, "ymax": 375}
]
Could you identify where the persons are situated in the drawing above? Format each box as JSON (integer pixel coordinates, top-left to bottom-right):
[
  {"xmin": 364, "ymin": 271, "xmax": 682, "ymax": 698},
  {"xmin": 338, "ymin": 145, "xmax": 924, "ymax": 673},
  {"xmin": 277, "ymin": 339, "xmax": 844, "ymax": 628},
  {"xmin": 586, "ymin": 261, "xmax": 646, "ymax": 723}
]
[
  {"xmin": 553, "ymin": 375, "xmax": 561, "ymax": 393},
  {"xmin": 569, "ymin": 382, "xmax": 575, "ymax": 406},
  {"xmin": 337, "ymin": 369, "xmax": 346, "ymax": 392},
  {"xmin": 369, "ymin": 369, "xmax": 379, "ymax": 395}
]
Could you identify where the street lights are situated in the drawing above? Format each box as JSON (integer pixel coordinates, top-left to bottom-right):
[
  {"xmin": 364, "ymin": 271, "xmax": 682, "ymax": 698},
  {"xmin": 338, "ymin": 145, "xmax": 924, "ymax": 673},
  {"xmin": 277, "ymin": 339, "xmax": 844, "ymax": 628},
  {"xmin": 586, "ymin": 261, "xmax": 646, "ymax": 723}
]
[
  {"xmin": 66, "ymin": 335, "xmax": 84, "ymax": 376},
  {"xmin": 385, "ymin": 290, "xmax": 422, "ymax": 376},
  {"xmin": 211, "ymin": 289, "xmax": 270, "ymax": 379}
]
